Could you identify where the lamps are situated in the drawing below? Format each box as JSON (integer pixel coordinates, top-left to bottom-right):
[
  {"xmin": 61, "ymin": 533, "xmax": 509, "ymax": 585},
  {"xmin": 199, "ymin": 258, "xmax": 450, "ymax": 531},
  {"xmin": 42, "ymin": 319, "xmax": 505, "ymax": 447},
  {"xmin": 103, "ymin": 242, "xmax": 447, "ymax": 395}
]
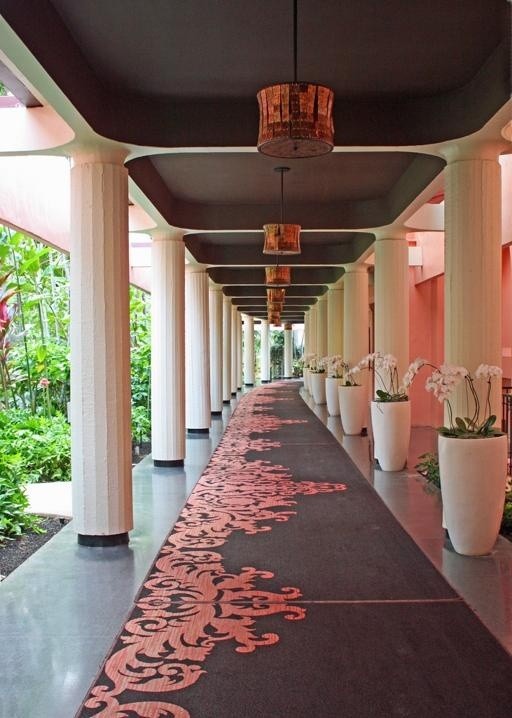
[
  {"xmin": 264, "ymin": 256, "xmax": 290, "ymax": 326},
  {"xmin": 255, "ymin": 1, "xmax": 336, "ymax": 158},
  {"xmin": 262, "ymin": 166, "xmax": 302, "ymax": 255}
]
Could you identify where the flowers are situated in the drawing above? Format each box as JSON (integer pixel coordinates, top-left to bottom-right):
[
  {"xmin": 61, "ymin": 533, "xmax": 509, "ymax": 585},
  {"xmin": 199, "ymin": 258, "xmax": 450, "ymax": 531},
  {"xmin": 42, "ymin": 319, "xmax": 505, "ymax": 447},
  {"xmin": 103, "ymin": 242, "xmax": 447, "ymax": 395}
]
[
  {"xmin": 347, "ymin": 352, "xmax": 439, "ymax": 400},
  {"xmin": 302, "ymin": 349, "xmax": 379, "ymax": 386},
  {"xmin": 425, "ymin": 362, "xmax": 502, "ymax": 436}
]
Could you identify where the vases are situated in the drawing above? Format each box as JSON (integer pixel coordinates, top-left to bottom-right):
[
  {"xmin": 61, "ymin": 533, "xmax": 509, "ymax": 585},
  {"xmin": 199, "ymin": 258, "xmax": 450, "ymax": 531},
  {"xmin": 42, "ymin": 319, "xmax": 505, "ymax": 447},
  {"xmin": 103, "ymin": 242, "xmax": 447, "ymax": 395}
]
[{"xmin": 302, "ymin": 368, "xmax": 344, "ymax": 416}]
